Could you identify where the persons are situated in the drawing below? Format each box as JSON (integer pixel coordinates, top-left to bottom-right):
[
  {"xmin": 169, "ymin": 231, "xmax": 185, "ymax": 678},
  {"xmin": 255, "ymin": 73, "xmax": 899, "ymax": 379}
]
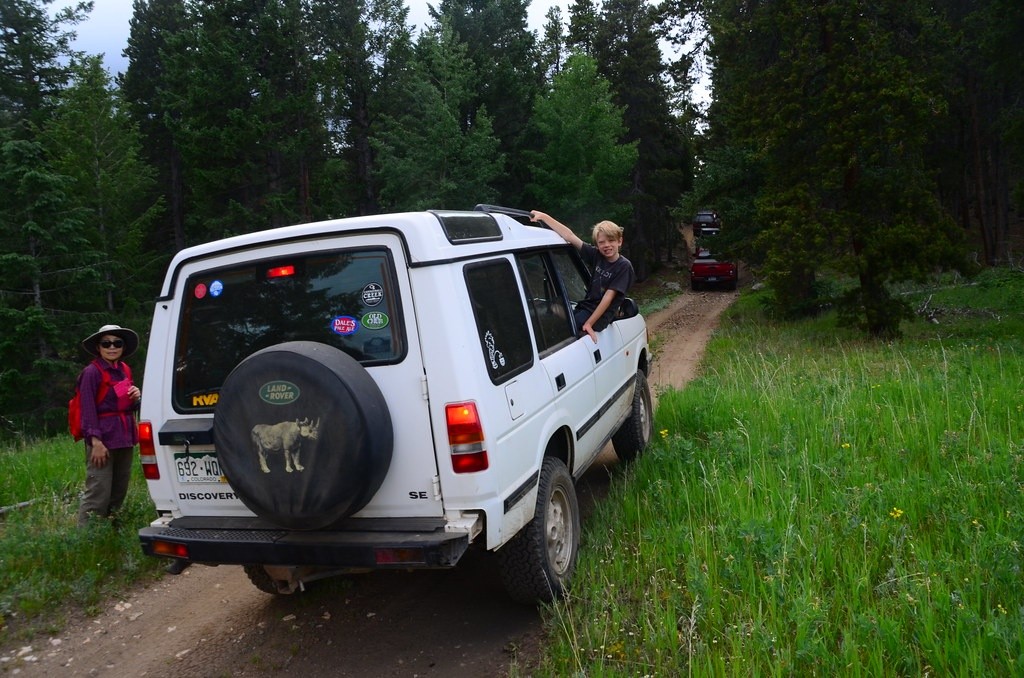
[
  {"xmin": 530, "ymin": 210, "xmax": 633, "ymax": 345},
  {"xmin": 78, "ymin": 325, "xmax": 141, "ymax": 528}
]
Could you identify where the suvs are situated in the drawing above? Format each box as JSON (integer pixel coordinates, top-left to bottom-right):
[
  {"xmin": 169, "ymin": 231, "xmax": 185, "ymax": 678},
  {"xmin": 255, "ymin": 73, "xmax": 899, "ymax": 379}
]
[
  {"xmin": 137, "ymin": 202, "xmax": 656, "ymax": 616},
  {"xmin": 690, "ymin": 246, "xmax": 739, "ymax": 291},
  {"xmin": 693, "ymin": 210, "xmax": 721, "ymax": 236}
]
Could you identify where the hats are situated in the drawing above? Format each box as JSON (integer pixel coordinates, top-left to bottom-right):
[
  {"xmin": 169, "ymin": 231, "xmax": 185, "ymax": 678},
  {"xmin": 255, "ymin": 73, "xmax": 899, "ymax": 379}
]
[{"xmin": 81, "ymin": 324, "xmax": 141, "ymax": 360}]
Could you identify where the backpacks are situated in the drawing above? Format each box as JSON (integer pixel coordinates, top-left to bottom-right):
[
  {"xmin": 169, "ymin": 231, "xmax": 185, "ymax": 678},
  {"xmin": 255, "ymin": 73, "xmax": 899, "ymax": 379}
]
[{"xmin": 69, "ymin": 361, "xmax": 132, "ymax": 437}]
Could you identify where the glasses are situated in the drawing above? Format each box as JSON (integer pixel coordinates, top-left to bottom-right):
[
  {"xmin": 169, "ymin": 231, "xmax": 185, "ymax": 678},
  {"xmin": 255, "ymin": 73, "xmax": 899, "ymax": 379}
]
[{"xmin": 95, "ymin": 339, "xmax": 126, "ymax": 349}]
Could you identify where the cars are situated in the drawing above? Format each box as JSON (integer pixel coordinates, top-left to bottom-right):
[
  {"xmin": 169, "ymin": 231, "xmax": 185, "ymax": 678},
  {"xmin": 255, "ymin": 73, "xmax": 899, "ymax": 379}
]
[{"xmin": 700, "ymin": 228, "xmax": 721, "ymax": 240}]
